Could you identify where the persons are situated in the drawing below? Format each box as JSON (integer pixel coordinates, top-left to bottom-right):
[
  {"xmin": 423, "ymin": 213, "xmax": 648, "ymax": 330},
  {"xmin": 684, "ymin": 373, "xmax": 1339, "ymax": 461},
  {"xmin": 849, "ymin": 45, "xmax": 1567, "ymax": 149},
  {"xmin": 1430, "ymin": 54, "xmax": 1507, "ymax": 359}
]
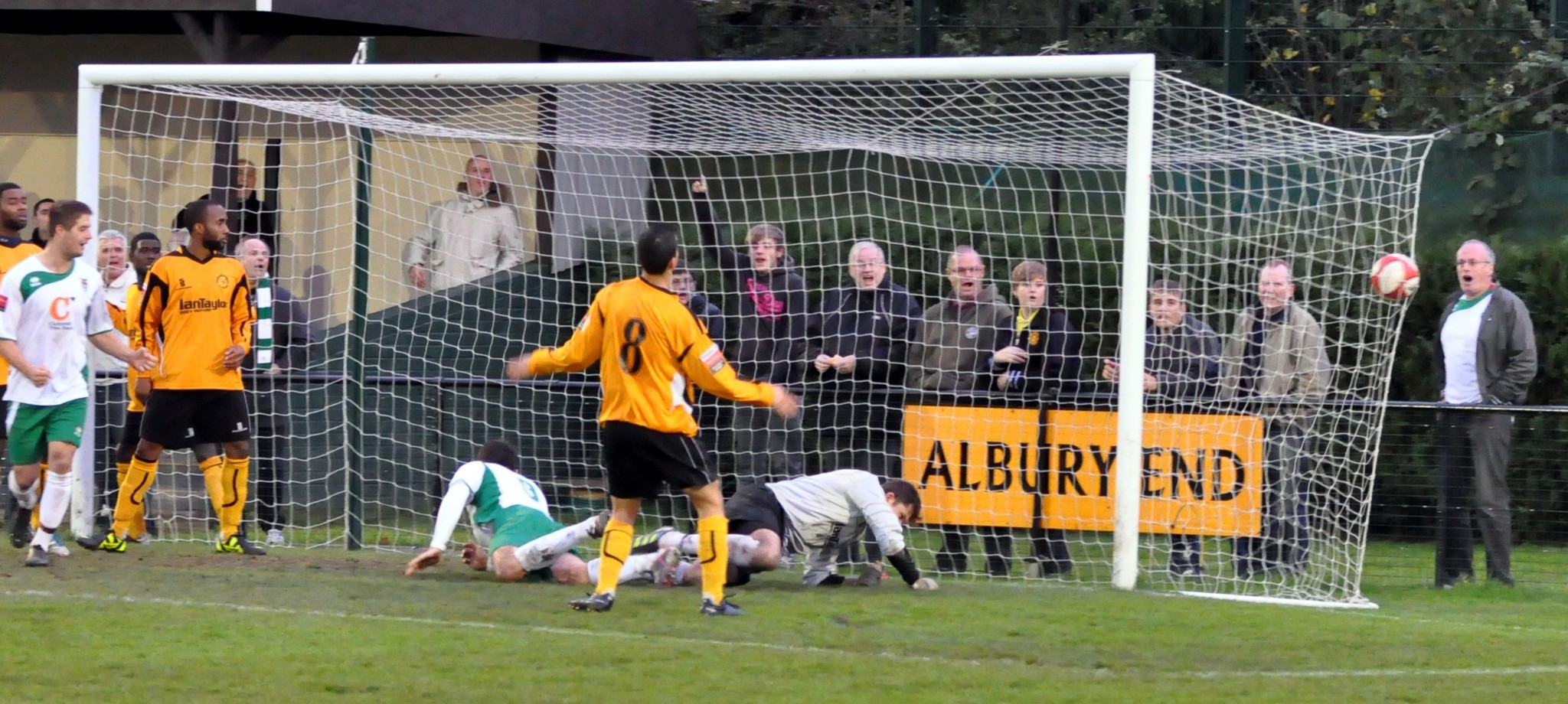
[
  {"xmin": 0, "ymin": 160, "xmax": 317, "ymax": 568},
  {"xmin": 806, "ymin": 240, "xmax": 920, "ymax": 564},
  {"xmin": 691, "ymin": 175, "xmax": 806, "ymax": 490},
  {"xmin": 629, "ymin": 468, "xmax": 939, "ymax": 592},
  {"xmin": 1101, "ymin": 279, "xmax": 1225, "ymax": 582},
  {"xmin": 1219, "ymin": 258, "xmax": 1331, "ymax": 575},
  {"xmin": 659, "ymin": 258, "xmax": 725, "ymax": 533},
  {"xmin": 981, "ymin": 261, "xmax": 1078, "ymax": 579},
  {"xmin": 1434, "ymin": 238, "xmax": 1538, "ymax": 586},
  {"xmin": 907, "ymin": 245, "xmax": 1012, "ymax": 578},
  {"xmin": 508, "ymin": 226, "xmax": 798, "ymax": 617},
  {"xmin": 403, "ymin": 441, "xmax": 680, "ymax": 589},
  {"xmin": 402, "ymin": 155, "xmax": 525, "ymax": 290}
]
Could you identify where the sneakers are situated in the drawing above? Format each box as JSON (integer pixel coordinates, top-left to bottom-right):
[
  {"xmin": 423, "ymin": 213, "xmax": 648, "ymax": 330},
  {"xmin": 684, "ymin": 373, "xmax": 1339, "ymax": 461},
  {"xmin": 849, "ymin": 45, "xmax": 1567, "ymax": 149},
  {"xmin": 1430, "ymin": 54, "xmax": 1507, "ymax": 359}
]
[
  {"xmin": 569, "ymin": 592, "xmax": 614, "ymax": 613},
  {"xmin": 75, "ymin": 528, "xmax": 127, "ymax": 553},
  {"xmin": 700, "ymin": 595, "xmax": 747, "ymax": 616},
  {"xmin": 216, "ymin": 534, "xmax": 266, "ymax": 555},
  {"xmin": 124, "ymin": 534, "xmax": 151, "ymax": 545}
]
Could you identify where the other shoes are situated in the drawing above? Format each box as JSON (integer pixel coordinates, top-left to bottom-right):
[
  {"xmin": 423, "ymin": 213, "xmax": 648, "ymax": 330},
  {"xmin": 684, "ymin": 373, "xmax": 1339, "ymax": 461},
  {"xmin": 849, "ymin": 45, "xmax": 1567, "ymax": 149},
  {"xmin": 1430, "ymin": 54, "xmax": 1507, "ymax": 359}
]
[
  {"xmin": 652, "ymin": 546, "xmax": 680, "ymax": 589},
  {"xmin": 24, "ymin": 546, "xmax": 49, "ymax": 565},
  {"xmin": 631, "ymin": 525, "xmax": 676, "ymax": 554},
  {"xmin": 265, "ymin": 529, "xmax": 284, "ymax": 547},
  {"xmin": 11, "ymin": 507, "xmax": 32, "ymax": 548},
  {"xmin": 589, "ymin": 509, "xmax": 612, "ymax": 538},
  {"xmin": 48, "ymin": 536, "xmax": 70, "ymax": 558}
]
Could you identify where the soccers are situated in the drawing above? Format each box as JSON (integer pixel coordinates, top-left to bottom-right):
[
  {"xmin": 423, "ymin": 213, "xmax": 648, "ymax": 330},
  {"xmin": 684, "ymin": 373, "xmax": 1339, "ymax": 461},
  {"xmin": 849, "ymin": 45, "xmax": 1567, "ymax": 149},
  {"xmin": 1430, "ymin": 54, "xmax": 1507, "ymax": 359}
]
[{"xmin": 1371, "ymin": 253, "xmax": 1419, "ymax": 300}]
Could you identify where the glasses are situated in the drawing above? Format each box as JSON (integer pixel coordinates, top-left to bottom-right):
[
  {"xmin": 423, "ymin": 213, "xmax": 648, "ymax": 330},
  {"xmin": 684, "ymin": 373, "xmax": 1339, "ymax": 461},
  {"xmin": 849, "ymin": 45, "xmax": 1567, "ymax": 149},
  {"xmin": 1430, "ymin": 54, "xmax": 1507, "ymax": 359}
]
[
  {"xmin": 1458, "ymin": 260, "xmax": 1491, "ymax": 267},
  {"xmin": 672, "ymin": 278, "xmax": 697, "ymax": 286}
]
